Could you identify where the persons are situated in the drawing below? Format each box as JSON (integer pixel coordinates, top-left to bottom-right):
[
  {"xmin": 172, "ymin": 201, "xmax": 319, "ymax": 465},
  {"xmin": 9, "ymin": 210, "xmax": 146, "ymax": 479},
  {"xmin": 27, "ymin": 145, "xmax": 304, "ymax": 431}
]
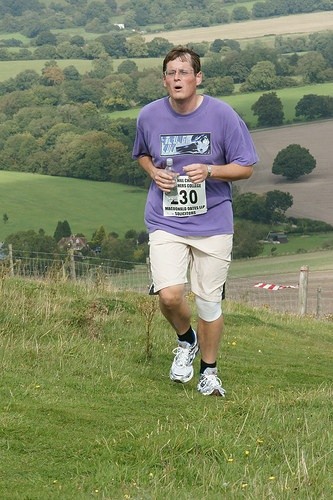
[{"xmin": 133, "ymin": 45, "xmax": 259, "ymax": 397}]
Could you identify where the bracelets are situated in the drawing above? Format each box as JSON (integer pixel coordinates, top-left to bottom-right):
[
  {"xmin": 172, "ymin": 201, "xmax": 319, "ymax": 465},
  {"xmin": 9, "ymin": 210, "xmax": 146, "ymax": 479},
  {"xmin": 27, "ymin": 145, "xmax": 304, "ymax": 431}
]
[{"xmin": 206, "ymin": 164, "xmax": 212, "ymax": 179}]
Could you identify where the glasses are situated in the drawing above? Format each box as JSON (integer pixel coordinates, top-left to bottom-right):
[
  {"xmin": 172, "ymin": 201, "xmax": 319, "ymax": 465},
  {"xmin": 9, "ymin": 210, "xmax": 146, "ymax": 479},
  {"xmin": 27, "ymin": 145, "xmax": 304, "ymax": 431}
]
[{"xmin": 163, "ymin": 68, "xmax": 195, "ymax": 77}]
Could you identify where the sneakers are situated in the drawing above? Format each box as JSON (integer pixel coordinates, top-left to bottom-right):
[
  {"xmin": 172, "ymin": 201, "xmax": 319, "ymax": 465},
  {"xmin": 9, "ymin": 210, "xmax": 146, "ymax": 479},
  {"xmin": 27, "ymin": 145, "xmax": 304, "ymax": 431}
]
[
  {"xmin": 170, "ymin": 329, "xmax": 200, "ymax": 384},
  {"xmin": 196, "ymin": 366, "xmax": 226, "ymax": 398}
]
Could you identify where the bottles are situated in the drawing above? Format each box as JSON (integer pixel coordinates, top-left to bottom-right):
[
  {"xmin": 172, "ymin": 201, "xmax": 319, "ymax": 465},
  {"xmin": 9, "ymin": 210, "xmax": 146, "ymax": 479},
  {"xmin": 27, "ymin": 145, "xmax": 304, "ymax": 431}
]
[{"xmin": 162, "ymin": 157, "xmax": 179, "ymax": 199}]
